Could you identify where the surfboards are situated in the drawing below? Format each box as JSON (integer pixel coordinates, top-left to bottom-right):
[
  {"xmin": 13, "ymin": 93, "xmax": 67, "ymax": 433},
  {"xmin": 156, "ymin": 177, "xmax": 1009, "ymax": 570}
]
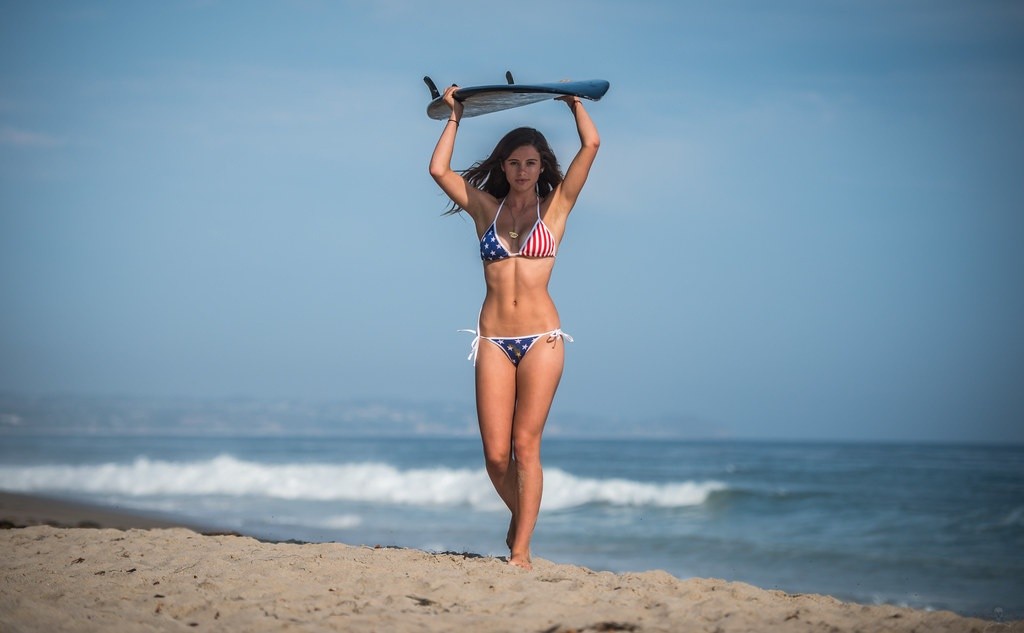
[{"xmin": 422, "ymin": 71, "xmax": 612, "ymax": 121}]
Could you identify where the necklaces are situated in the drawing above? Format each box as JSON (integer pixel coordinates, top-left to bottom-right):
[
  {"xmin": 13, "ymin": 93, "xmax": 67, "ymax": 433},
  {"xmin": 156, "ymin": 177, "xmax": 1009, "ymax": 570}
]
[{"xmin": 504, "ymin": 190, "xmax": 540, "ymax": 240}]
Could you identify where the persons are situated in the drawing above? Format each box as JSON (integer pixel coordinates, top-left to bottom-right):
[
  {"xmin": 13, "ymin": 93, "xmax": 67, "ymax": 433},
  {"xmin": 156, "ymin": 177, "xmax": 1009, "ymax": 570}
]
[{"xmin": 429, "ymin": 78, "xmax": 601, "ymax": 572}]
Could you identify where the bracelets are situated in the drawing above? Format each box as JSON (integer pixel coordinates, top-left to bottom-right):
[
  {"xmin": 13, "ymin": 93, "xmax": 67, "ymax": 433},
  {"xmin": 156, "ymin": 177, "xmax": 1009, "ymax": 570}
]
[{"xmin": 447, "ymin": 118, "xmax": 459, "ymax": 126}]
